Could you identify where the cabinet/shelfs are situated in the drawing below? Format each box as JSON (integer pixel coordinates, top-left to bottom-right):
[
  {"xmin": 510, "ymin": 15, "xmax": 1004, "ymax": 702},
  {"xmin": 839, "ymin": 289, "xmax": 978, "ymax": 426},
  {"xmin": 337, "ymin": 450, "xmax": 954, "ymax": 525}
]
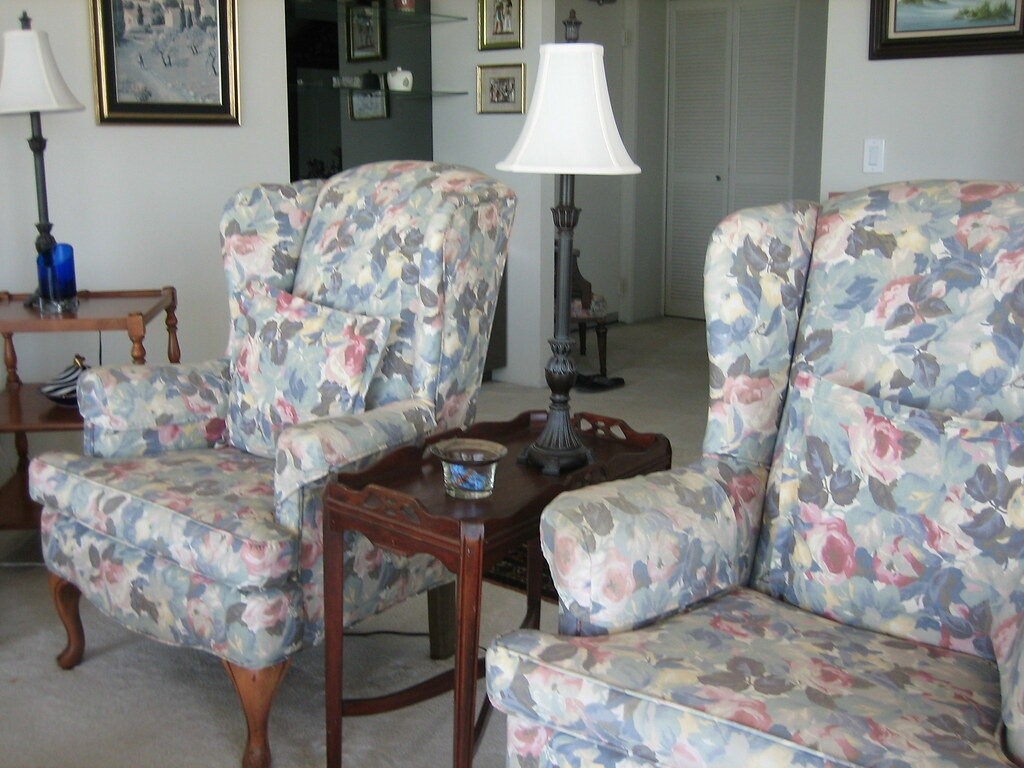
[{"xmin": 294, "ymin": 6, "xmax": 469, "ymax": 101}]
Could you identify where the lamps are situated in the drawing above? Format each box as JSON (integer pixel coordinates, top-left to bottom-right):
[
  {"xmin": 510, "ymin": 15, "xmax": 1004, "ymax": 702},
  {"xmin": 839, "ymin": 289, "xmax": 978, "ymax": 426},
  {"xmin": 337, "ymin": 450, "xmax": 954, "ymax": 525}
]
[
  {"xmin": 495, "ymin": 8, "xmax": 644, "ymax": 478},
  {"xmin": 0, "ymin": 11, "xmax": 89, "ymax": 314}
]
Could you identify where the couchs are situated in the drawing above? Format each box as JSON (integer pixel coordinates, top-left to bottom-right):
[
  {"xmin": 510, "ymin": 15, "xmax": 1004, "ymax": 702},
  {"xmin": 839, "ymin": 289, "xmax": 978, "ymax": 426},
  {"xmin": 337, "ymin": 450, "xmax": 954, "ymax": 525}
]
[
  {"xmin": 28, "ymin": 160, "xmax": 517, "ymax": 768},
  {"xmin": 484, "ymin": 178, "xmax": 1024, "ymax": 768}
]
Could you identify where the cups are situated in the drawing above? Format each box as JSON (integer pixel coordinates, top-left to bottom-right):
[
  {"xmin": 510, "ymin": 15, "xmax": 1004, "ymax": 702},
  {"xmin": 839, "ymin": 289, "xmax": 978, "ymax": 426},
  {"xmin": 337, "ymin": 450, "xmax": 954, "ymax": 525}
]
[
  {"xmin": 429, "ymin": 438, "xmax": 508, "ymax": 500},
  {"xmin": 37, "ymin": 243, "xmax": 80, "ymax": 312}
]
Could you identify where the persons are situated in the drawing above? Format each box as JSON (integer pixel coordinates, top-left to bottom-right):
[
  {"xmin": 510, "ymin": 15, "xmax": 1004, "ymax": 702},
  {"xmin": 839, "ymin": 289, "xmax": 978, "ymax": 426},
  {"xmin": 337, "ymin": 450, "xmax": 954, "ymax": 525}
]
[{"xmin": 494, "ymin": 0, "xmax": 512, "ymax": 33}]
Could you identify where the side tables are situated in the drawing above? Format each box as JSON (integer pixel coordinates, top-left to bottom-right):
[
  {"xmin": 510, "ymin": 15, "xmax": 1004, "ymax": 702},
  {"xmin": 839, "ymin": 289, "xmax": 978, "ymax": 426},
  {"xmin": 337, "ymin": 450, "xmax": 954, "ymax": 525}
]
[
  {"xmin": 0, "ymin": 286, "xmax": 181, "ymax": 530},
  {"xmin": 319, "ymin": 410, "xmax": 673, "ymax": 768}
]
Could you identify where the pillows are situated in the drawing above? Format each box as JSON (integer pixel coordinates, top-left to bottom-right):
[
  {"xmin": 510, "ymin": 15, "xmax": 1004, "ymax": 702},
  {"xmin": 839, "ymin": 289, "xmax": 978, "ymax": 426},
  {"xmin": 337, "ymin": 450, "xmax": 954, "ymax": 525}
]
[
  {"xmin": 749, "ymin": 368, "xmax": 1024, "ymax": 657},
  {"xmin": 219, "ymin": 275, "xmax": 394, "ymax": 455}
]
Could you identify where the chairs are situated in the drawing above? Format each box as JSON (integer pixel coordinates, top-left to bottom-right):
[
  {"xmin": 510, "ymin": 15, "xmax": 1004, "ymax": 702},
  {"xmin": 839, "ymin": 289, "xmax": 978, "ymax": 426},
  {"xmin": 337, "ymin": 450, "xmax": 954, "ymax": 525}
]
[{"xmin": 554, "ymin": 249, "xmax": 625, "ymax": 392}]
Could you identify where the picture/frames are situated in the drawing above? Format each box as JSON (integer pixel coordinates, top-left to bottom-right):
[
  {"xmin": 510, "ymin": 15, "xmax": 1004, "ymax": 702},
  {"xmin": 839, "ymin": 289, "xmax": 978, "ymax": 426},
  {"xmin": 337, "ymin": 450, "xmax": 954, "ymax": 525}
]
[
  {"xmin": 343, "ymin": 0, "xmax": 388, "ymax": 64},
  {"xmin": 346, "ymin": 88, "xmax": 391, "ymax": 122},
  {"xmin": 87, "ymin": 0, "xmax": 244, "ymax": 128},
  {"xmin": 475, "ymin": 62, "xmax": 526, "ymax": 116},
  {"xmin": 866, "ymin": 0, "xmax": 1024, "ymax": 63},
  {"xmin": 475, "ymin": 0, "xmax": 524, "ymax": 53}
]
